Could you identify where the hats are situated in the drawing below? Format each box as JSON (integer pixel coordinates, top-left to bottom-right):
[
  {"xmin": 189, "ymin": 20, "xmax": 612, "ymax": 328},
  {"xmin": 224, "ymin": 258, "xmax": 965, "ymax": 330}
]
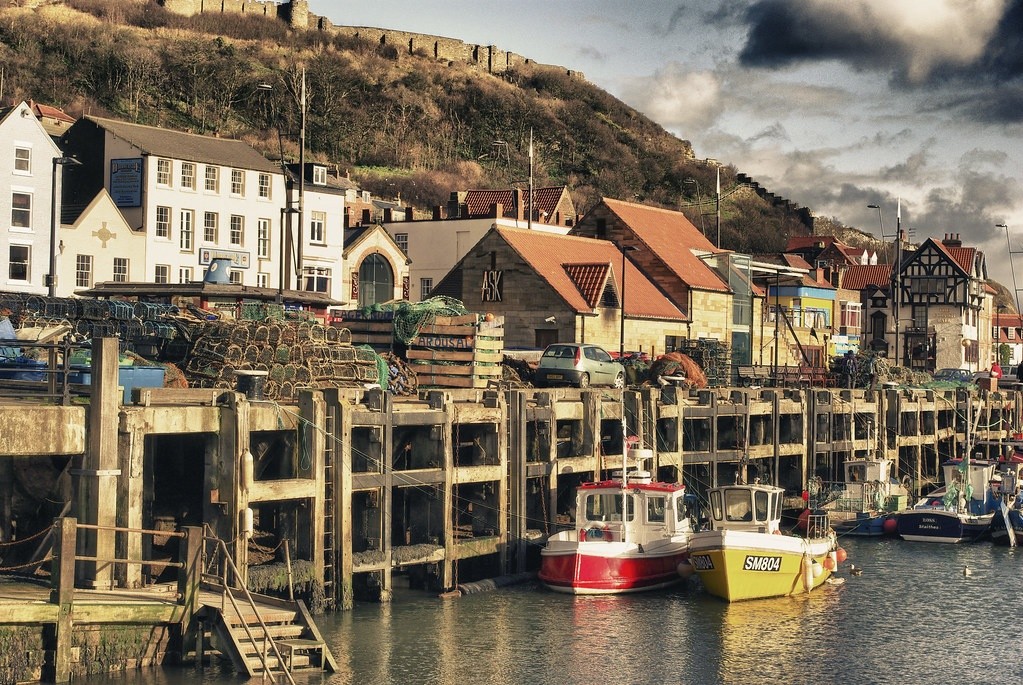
[{"xmin": 992, "ymin": 362, "xmax": 996, "ymax": 365}]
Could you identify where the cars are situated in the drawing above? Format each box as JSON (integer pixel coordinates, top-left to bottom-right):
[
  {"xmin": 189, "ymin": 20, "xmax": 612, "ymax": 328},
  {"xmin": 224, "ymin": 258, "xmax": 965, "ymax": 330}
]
[
  {"xmin": 961, "ymin": 372, "xmax": 990, "ymax": 383},
  {"xmin": 535, "ymin": 343, "xmax": 626, "ymax": 389},
  {"xmin": 1001, "ymin": 366, "xmax": 1018, "ymax": 380},
  {"xmin": 932, "ymin": 368, "xmax": 971, "ymax": 382}
]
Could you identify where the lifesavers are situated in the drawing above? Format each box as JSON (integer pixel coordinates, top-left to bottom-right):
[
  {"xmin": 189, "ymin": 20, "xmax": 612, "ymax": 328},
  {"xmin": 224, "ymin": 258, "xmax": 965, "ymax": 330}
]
[
  {"xmin": 902, "ymin": 475, "xmax": 912, "ymax": 490},
  {"xmin": 579, "ymin": 521, "xmax": 612, "ymax": 542},
  {"xmin": 627, "ymin": 434, "xmax": 639, "ymax": 449}
]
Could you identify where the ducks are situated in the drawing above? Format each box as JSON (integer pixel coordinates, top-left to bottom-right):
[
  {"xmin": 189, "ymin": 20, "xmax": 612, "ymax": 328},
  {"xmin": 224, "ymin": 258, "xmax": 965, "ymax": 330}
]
[
  {"xmin": 963, "ymin": 565, "xmax": 972, "ymax": 576},
  {"xmin": 849, "ymin": 564, "xmax": 863, "ymax": 576}
]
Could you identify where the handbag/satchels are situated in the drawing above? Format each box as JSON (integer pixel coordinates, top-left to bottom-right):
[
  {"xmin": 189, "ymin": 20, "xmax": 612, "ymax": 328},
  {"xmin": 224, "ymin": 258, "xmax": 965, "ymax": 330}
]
[{"xmin": 991, "ymin": 370, "xmax": 998, "ymax": 377}]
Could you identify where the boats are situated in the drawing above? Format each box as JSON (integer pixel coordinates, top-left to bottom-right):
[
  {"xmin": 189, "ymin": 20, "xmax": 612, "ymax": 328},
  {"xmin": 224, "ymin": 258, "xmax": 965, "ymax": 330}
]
[
  {"xmin": 538, "ymin": 416, "xmax": 709, "ymax": 594},
  {"xmin": 989, "ymin": 468, "xmax": 1023, "ymax": 548},
  {"xmin": 688, "ymin": 477, "xmax": 837, "ymax": 604},
  {"xmin": 808, "ymin": 421, "xmax": 913, "ymax": 535},
  {"xmin": 897, "ymin": 398, "xmax": 1002, "ymax": 543}
]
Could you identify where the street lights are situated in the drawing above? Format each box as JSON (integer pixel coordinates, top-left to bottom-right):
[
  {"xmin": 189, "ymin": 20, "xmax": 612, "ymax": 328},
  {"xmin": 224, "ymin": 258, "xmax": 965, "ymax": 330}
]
[
  {"xmin": 867, "ymin": 204, "xmax": 901, "ymax": 366},
  {"xmin": 683, "ymin": 179, "xmax": 720, "ymax": 249},
  {"xmin": 621, "ymin": 245, "xmax": 641, "ymax": 357},
  {"xmin": 491, "ymin": 141, "xmax": 532, "ymax": 230},
  {"xmin": 865, "ymin": 284, "xmax": 881, "ymax": 350},
  {"xmin": 774, "ymin": 268, "xmax": 788, "ymax": 388},
  {"xmin": 257, "ymin": 85, "xmax": 305, "ymax": 290},
  {"xmin": 996, "ymin": 224, "xmax": 1022, "ymax": 325},
  {"xmin": 997, "ymin": 304, "xmax": 1007, "ymax": 365},
  {"xmin": 48, "ymin": 156, "xmax": 82, "ymax": 299}
]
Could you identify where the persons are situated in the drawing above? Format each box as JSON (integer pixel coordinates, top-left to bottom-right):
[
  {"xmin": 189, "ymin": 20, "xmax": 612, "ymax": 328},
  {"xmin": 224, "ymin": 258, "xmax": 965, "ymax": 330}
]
[
  {"xmin": 990, "ymin": 362, "xmax": 1002, "ymax": 390},
  {"xmin": 842, "ymin": 350, "xmax": 859, "ymax": 389},
  {"xmin": 869, "ymin": 354, "xmax": 878, "ymax": 394},
  {"xmin": 1016, "ymin": 361, "xmax": 1023, "ymax": 382}
]
